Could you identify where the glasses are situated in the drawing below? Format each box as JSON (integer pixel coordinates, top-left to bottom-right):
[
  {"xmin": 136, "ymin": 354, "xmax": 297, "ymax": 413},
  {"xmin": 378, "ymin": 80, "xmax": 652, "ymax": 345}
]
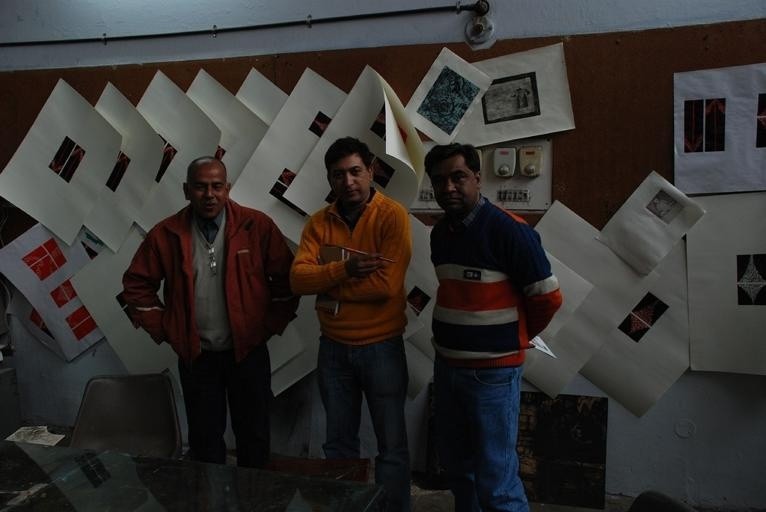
[{"xmin": 207, "ymin": 242, "xmax": 217, "ymax": 275}]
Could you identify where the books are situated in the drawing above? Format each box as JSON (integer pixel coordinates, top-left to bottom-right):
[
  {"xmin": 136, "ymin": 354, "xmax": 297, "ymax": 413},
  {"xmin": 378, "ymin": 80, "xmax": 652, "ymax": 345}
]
[{"xmin": 316, "ymin": 246, "xmax": 350, "ymax": 314}]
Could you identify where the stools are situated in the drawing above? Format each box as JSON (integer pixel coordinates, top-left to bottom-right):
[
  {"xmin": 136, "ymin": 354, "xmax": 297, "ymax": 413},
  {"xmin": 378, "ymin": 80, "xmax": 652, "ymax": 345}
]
[{"xmin": 270, "ymin": 456, "xmax": 373, "ymax": 484}]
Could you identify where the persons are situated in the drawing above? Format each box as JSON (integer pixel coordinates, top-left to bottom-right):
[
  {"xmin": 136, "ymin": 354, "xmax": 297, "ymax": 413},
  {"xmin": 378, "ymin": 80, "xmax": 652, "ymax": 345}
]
[
  {"xmin": 510, "ymin": 86, "xmax": 530, "ymax": 110},
  {"xmin": 289, "ymin": 137, "xmax": 412, "ymax": 512},
  {"xmin": 122, "ymin": 155, "xmax": 300, "ymax": 468},
  {"xmin": 653, "ymin": 196, "xmax": 674, "ymax": 217},
  {"xmin": 425, "ymin": 143, "xmax": 563, "ymax": 511}
]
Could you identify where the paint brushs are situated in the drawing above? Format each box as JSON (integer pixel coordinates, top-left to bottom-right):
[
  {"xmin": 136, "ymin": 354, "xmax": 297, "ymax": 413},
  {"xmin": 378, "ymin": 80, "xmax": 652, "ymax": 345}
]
[{"xmin": 337, "ymin": 245, "xmax": 396, "ymax": 264}]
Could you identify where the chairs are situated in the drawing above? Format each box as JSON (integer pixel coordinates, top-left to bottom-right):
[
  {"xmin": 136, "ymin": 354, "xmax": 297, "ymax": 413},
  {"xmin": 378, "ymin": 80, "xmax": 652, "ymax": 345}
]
[{"xmin": 68, "ymin": 370, "xmax": 184, "ymax": 461}]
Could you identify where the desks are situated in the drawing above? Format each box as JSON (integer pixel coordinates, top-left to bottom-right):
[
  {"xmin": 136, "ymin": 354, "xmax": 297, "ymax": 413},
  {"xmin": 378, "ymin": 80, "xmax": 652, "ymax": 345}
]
[{"xmin": 0, "ymin": 438, "xmax": 387, "ymax": 512}]
